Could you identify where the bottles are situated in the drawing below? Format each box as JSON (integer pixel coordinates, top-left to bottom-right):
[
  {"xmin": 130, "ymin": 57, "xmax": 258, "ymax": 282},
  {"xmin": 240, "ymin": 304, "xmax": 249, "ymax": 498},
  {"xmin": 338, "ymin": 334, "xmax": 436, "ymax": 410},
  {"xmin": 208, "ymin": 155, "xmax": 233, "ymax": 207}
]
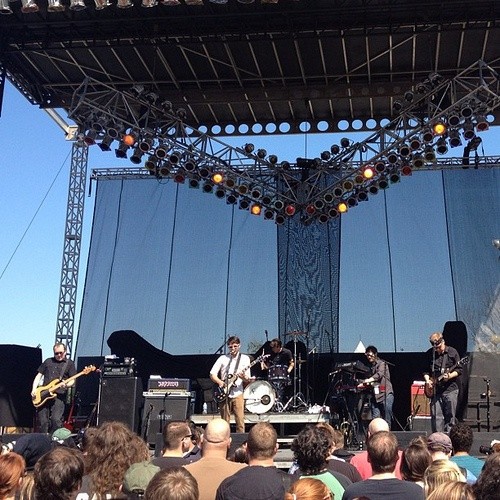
[{"xmin": 202, "ymin": 402, "xmax": 207, "ymax": 415}]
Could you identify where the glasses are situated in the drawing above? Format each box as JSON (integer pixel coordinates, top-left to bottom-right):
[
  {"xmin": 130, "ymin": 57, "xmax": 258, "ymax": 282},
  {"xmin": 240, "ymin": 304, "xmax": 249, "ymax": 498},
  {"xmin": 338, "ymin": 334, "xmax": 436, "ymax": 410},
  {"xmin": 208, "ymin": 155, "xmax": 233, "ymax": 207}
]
[
  {"xmin": 181, "ymin": 434, "xmax": 195, "ymax": 441},
  {"xmin": 229, "ymin": 346, "xmax": 237, "ymax": 348},
  {"xmin": 54, "ymin": 352, "xmax": 62, "ymax": 355}
]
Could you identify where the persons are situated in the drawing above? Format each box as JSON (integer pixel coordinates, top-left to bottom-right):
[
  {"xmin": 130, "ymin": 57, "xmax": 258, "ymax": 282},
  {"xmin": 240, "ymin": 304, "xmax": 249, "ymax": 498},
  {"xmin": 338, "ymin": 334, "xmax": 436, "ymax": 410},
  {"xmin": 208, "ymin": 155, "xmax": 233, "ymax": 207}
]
[
  {"xmin": 210, "ymin": 336, "xmax": 255, "ymax": 433},
  {"xmin": 261, "ymin": 339, "xmax": 294, "ymax": 377},
  {"xmin": 31, "ymin": 343, "xmax": 77, "ymax": 434},
  {"xmin": 421, "ymin": 332, "xmax": 463, "ymax": 434},
  {"xmin": 0, "ymin": 418, "xmax": 500, "ymax": 500},
  {"xmin": 362, "ymin": 346, "xmax": 394, "ymax": 431}
]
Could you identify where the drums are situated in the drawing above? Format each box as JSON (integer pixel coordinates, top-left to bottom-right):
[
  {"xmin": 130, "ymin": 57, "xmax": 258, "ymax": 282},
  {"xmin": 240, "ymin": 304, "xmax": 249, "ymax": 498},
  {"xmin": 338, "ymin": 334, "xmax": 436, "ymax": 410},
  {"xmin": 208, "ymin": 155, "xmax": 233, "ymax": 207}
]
[{"xmin": 243, "ymin": 364, "xmax": 291, "ymax": 413}]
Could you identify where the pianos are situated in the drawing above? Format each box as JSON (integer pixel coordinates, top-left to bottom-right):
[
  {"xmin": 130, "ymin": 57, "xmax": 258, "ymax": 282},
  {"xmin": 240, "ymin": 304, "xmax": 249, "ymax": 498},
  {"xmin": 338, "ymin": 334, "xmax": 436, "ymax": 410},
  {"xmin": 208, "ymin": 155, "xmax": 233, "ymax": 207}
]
[{"xmin": 334, "ymin": 381, "xmax": 387, "ymax": 447}]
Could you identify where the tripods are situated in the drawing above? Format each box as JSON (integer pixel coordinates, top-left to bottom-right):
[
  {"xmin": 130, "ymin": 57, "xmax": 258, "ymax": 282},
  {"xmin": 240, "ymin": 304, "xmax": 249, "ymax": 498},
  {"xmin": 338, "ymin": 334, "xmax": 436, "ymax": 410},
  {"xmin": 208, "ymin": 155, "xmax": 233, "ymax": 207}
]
[{"xmin": 271, "ymin": 333, "xmax": 310, "ymax": 414}]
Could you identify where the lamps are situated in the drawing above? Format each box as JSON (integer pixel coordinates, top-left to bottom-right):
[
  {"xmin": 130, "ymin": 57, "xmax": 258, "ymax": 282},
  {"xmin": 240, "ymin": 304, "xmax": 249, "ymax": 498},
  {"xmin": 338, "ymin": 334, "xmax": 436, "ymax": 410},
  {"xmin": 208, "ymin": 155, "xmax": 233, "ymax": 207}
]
[
  {"xmin": 297, "ymin": 72, "xmax": 489, "ymax": 227},
  {"xmin": 0, "ymin": 0, "xmax": 277, "ymax": 15},
  {"xmin": 127, "ymin": 83, "xmax": 188, "ymax": 121},
  {"xmin": 77, "ymin": 123, "xmax": 295, "ymax": 226}
]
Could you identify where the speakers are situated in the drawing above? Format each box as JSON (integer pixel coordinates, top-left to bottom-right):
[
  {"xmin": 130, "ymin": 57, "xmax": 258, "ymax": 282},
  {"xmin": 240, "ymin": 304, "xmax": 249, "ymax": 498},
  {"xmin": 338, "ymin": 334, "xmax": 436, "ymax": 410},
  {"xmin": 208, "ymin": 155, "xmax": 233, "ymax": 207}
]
[
  {"xmin": 226, "ymin": 433, "xmax": 248, "ymax": 461},
  {"xmin": 412, "ymin": 417, "xmax": 444, "ymax": 438},
  {"xmin": 155, "ymin": 433, "xmax": 164, "ymax": 457},
  {"xmin": 411, "ymin": 384, "xmax": 432, "ymax": 416},
  {"xmin": 143, "ymin": 397, "xmax": 196, "ymax": 444},
  {"xmin": 96, "ymin": 377, "xmax": 143, "ymax": 436},
  {"xmin": 0, "ymin": 344, "xmax": 42, "ymax": 428},
  {"xmin": 391, "ymin": 430, "xmax": 427, "ymax": 450}
]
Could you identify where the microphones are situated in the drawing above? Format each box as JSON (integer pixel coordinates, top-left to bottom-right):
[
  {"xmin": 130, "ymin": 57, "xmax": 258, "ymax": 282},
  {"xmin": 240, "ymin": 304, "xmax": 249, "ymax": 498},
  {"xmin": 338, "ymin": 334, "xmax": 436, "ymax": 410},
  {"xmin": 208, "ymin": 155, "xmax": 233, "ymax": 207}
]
[{"xmin": 308, "ymin": 346, "xmax": 318, "ymax": 354}]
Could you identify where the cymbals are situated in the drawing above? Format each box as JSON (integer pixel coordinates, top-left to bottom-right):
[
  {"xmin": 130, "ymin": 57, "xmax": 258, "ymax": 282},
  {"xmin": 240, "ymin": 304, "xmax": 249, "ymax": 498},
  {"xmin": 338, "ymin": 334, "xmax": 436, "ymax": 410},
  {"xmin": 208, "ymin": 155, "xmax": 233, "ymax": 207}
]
[{"xmin": 281, "ymin": 331, "xmax": 308, "ymax": 336}]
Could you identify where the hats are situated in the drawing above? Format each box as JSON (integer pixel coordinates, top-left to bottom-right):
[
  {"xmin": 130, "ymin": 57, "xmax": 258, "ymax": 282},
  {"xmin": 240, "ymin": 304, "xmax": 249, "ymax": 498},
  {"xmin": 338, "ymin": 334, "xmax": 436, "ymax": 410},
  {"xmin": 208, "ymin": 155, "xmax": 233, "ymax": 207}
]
[
  {"xmin": 51, "ymin": 428, "xmax": 78, "ymax": 440},
  {"xmin": 428, "ymin": 433, "xmax": 453, "ymax": 450},
  {"xmin": 14, "ymin": 434, "xmax": 59, "ymax": 472},
  {"xmin": 124, "ymin": 461, "xmax": 161, "ymax": 494}
]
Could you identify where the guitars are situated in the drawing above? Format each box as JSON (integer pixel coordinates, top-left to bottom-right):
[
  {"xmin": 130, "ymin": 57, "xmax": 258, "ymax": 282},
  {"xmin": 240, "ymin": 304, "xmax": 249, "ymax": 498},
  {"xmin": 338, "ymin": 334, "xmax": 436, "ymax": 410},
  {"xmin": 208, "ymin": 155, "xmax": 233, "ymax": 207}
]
[
  {"xmin": 211, "ymin": 354, "xmax": 268, "ymax": 403},
  {"xmin": 424, "ymin": 356, "xmax": 469, "ymax": 399},
  {"xmin": 32, "ymin": 364, "xmax": 96, "ymax": 408}
]
